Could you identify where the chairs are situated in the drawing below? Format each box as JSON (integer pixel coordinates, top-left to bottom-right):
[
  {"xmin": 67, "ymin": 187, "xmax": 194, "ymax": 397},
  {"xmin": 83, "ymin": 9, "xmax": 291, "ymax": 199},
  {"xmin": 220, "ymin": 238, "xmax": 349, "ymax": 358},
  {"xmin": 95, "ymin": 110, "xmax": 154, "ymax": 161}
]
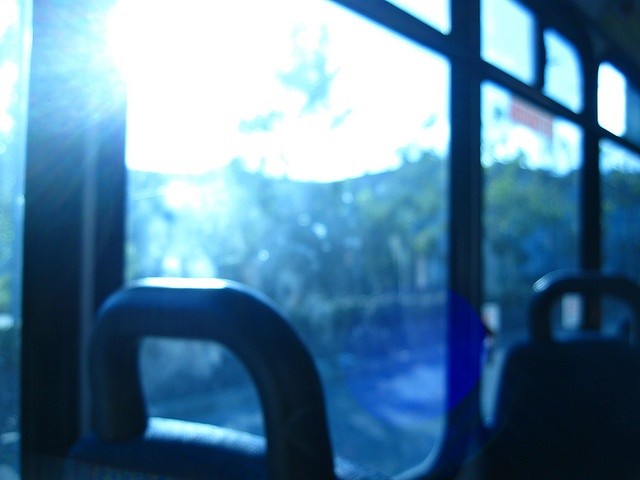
[
  {"xmin": 483, "ymin": 269, "xmax": 640, "ymax": 478},
  {"xmin": 65, "ymin": 290, "xmax": 337, "ymax": 476}
]
